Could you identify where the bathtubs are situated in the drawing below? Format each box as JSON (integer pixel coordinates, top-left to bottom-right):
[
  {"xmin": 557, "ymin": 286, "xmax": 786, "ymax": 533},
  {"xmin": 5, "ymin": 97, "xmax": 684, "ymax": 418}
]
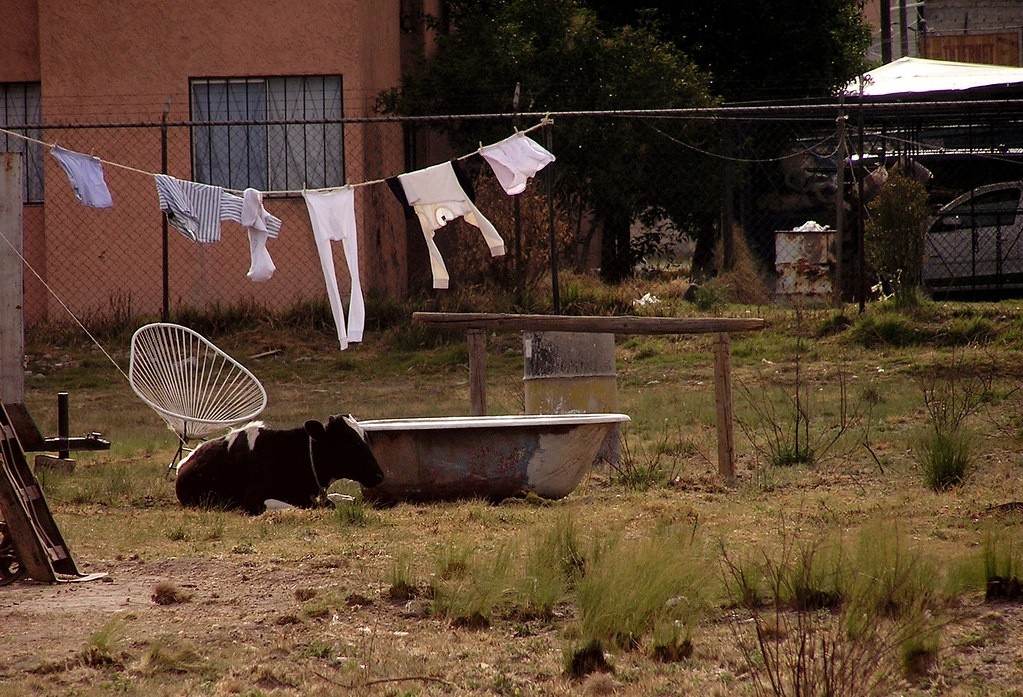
[{"xmin": 342, "ymin": 412, "xmax": 632, "ymax": 501}]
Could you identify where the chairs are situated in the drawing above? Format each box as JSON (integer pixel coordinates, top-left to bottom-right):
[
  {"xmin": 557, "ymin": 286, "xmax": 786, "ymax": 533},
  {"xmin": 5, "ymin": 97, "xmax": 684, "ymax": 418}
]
[{"xmin": 128, "ymin": 323, "xmax": 268, "ymax": 479}]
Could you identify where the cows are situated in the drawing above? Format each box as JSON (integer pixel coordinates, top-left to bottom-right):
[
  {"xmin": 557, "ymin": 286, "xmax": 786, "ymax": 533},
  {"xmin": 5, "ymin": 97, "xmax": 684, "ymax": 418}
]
[{"xmin": 175, "ymin": 411, "xmax": 386, "ymax": 517}]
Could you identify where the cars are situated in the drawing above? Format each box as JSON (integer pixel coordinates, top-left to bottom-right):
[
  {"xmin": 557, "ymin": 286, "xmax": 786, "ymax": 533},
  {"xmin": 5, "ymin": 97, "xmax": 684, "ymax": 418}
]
[{"xmin": 922, "ymin": 181, "xmax": 1023, "ymax": 286}]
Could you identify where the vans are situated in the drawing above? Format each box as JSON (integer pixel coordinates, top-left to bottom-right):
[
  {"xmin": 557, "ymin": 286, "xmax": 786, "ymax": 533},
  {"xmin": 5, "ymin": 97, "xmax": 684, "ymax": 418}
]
[{"xmin": 843, "ymin": 149, "xmax": 1023, "ymax": 226}]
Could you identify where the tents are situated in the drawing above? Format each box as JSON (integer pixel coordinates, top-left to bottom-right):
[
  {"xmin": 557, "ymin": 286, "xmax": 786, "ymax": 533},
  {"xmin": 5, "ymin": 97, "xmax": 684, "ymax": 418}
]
[{"xmin": 827, "ymin": 56, "xmax": 1023, "ymax": 172}]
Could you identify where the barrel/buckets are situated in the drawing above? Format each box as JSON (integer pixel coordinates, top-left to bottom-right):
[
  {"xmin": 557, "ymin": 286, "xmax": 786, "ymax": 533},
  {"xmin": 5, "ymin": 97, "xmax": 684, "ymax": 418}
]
[
  {"xmin": 776, "ymin": 229, "xmax": 837, "ymax": 309},
  {"xmin": 521, "ymin": 329, "xmax": 620, "ymax": 469}
]
